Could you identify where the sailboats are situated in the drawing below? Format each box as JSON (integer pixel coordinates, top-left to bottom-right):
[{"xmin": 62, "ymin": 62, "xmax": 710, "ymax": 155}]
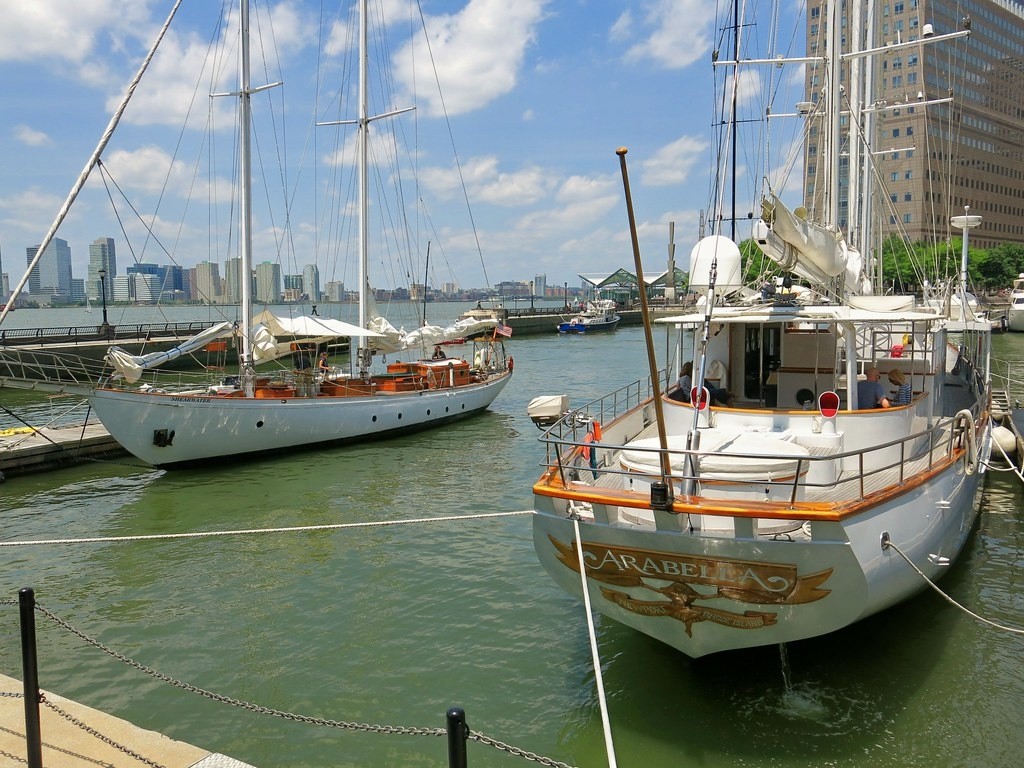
[
  {"xmin": 0, "ymin": 0, "xmax": 515, "ymax": 472},
  {"xmin": 531, "ymin": 1, "xmax": 994, "ymax": 668},
  {"xmin": 84, "ymin": 297, "xmax": 93, "ymax": 313}
]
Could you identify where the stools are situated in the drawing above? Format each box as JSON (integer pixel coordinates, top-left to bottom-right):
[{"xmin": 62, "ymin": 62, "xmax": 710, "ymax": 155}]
[{"xmin": 784, "ymin": 434, "xmax": 842, "ymax": 488}]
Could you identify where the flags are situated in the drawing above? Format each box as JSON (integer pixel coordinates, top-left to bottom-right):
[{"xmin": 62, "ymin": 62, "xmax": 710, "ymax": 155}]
[{"xmin": 496, "ymin": 323, "xmax": 512, "ymax": 338}]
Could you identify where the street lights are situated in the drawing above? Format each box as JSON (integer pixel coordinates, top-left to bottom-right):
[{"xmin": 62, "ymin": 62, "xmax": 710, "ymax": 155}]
[
  {"xmin": 530, "ymin": 281, "xmax": 533, "ymax": 307},
  {"xmin": 99, "ymin": 269, "xmax": 109, "ymax": 325},
  {"xmin": 565, "ymin": 282, "xmax": 567, "ymax": 306}
]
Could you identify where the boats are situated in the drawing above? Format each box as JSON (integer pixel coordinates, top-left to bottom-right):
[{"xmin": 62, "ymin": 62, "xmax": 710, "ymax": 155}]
[
  {"xmin": 559, "ymin": 285, "xmax": 621, "ymax": 334},
  {"xmin": 1008, "ymin": 272, "xmax": 1024, "ymax": 332}
]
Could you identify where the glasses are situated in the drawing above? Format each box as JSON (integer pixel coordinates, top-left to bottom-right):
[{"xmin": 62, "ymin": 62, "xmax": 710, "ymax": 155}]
[
  {"xmin": 889, "ymin": 378, "xmax": 894, "ymax": 382},
  {"xmin": 877, "ymin": 375, "xmax": 880, "ymax": 379}
]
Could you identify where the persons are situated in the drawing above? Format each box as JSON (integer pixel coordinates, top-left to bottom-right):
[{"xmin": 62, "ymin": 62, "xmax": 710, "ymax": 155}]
[
  {"xmin": 294, "ymin": 356, "xmax": 311, "ymax": 371},
  {"xmin": 432, "ymin": 346, "xmax": 446, "ymax": 358},
  {"xmin": 888, "ymin": 369, "xmax": 911, "ymax": 406},
  {"xmin": 858, "ymin": 368, "xmax": 890, "ymax": 409},
  {"xmin": 677, "ymin": 361, "xmax": 735, "ymax": 408},
  {"xmin": 319, "ymin": 352, "xmax": 332, "ymax": 374}
]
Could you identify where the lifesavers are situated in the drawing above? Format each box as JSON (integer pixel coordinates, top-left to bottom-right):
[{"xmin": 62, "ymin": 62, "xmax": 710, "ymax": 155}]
[
  {"xmin": 507, "ymin": 356, "xmax": 514, "ymax": 371},
  {"xmin": 572, "ymin": 321, "xmax": 576, "ymax": 324}
]
